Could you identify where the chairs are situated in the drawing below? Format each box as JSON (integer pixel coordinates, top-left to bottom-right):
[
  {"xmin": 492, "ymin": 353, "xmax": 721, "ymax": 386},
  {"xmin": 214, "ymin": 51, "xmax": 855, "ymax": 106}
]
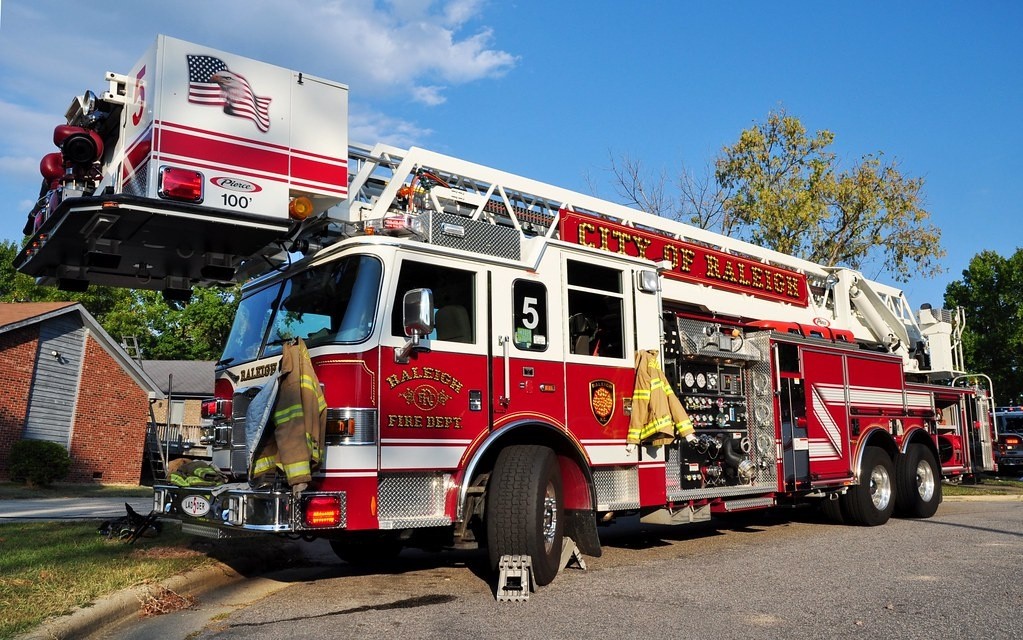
[{"xmin": 435, "ymin": 303, "xmax": 471, "ymax": 343}]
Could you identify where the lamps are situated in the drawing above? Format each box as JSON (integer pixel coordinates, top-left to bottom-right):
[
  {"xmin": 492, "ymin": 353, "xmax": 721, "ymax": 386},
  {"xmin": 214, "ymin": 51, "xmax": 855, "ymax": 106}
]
[{"xmin": 52, "ymin": 349, "xmax": 58, "ymax": 358}]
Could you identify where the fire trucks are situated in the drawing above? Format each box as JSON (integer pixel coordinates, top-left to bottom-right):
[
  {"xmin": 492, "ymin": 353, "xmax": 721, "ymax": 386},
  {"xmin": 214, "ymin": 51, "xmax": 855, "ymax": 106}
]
[
  {"xmin": 989, "ymin": 406, "xmax": 1023, "ymax": 467},
  {"xmin": 13, "ymin": 36, "xmax": 998, "ymax": 586}
]
